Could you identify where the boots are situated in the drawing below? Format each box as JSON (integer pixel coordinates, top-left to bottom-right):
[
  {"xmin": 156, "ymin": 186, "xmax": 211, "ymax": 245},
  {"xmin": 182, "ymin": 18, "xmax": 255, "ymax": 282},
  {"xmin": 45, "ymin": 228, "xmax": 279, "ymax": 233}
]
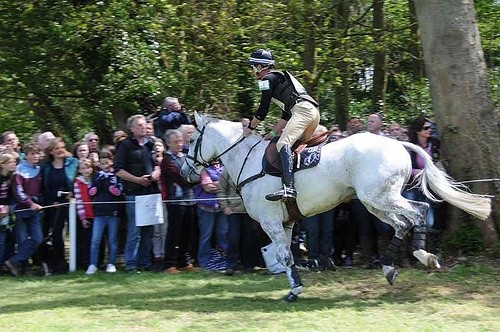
[{"xmin": 265, "ymin": 144, "xmax": 297, "ymax": 201}]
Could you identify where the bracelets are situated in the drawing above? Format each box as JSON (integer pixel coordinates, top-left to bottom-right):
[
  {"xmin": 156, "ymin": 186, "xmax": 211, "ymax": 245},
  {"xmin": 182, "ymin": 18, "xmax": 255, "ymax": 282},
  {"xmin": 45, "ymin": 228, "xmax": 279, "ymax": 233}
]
[{"xmin": 247, "ymin": 122, "xmax": 256, "ymax": 130}]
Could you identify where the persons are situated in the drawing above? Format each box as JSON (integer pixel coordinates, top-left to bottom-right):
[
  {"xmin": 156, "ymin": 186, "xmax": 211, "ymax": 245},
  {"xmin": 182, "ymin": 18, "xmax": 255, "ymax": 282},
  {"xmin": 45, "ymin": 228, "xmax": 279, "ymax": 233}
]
[
  {"xmin": 86, "ymin": 152, "xmax": 126, "ymax": 274},
  {"xmin": 4, "ymin": 144, "xmax": 44, "ymax": 278},
  {"xmin": 0, "ymin": 97, "xmax": 445, "ymax": 277},
  {"xmin": 0, "ymin": 153, "xmax": 17, "ymax": 273},
  {"xmin": 37, "ymin": 136, "xmax": 79, "ymax": 274},
  {"xmin": 112, "ymin": 115, "xmax": 161, "ymax": 273},
  {"xmin": 160, "ymin": 129, "xmax": 201, "ymax": 272},
  {"xmin": 197, "ymin": 159, "xmax": 229, "ymax": 274},
  {"xmin": 73, "ymin": 157, "xmax": 94, "ymax": 270},
  {"xmin": 241, "ymin": 48, "xmax": 321, "ymax": 203}
]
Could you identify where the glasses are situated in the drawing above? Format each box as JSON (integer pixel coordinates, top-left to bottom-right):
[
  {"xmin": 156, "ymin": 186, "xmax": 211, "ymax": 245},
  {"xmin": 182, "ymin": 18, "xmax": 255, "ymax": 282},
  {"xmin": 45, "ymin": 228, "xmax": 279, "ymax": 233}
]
[
  {"xmin": 422, "ymin": 126, "xmax": 430, "ymax": 130},
  {"xmin": 88, "ymin": 139, "xmax": 98, "ymax": 142}
]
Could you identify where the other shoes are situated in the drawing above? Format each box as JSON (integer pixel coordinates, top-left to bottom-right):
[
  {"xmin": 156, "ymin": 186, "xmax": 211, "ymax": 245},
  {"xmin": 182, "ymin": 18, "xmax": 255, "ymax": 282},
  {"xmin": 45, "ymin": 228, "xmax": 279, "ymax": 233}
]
[
  {"xmin": 295, "ymin": 239, "xmax": 427, "ymax": 272},
  {"xmin": 0, "ymin": 259, "xmax": 270, "ymax": 277}
]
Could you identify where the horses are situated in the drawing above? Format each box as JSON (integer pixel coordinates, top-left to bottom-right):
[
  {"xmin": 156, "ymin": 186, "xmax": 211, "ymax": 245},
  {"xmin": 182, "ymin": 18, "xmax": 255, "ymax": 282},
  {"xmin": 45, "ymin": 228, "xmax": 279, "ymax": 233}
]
[{"xmin": 180, "ymin": 109, "xmax": 494, "ymax": 301}]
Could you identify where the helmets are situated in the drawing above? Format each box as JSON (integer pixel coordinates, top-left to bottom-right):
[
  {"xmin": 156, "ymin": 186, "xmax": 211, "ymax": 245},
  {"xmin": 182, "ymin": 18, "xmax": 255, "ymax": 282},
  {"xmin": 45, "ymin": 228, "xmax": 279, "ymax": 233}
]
[{"xmin": 247, "ymin": 49, "xmax": 275, "ymax": 65}]
